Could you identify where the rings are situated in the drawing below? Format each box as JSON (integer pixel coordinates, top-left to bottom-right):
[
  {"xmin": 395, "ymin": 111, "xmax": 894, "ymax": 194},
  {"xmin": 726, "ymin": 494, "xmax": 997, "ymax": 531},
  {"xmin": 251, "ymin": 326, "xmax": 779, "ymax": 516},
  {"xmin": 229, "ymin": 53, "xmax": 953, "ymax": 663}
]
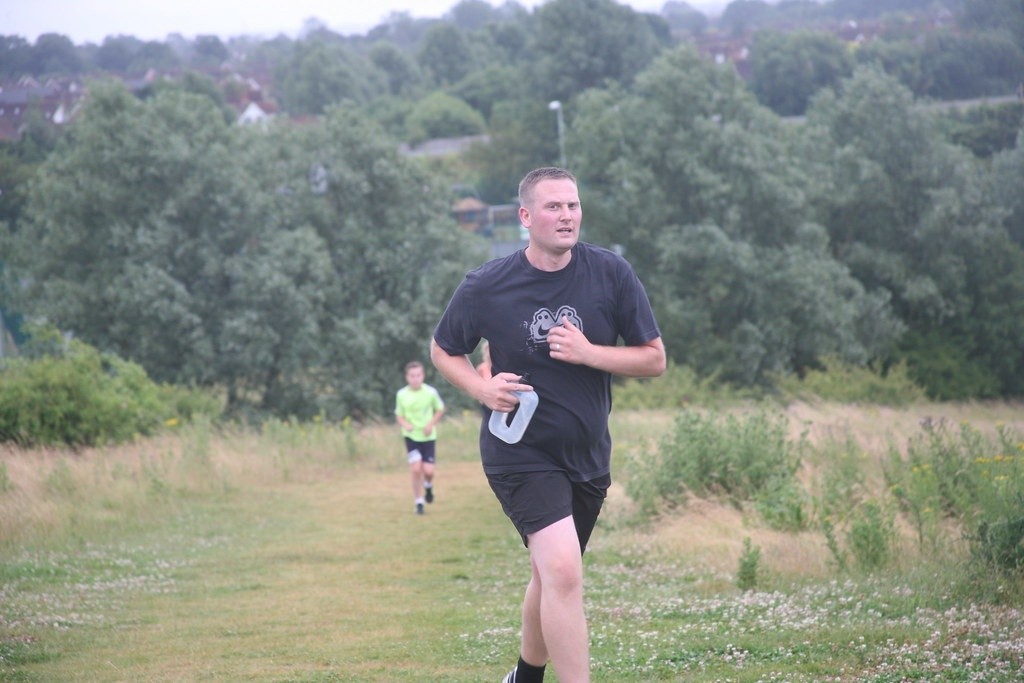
[{"xmin": 556, "ymin": 344, "xmax": 560, "ymax": 351}]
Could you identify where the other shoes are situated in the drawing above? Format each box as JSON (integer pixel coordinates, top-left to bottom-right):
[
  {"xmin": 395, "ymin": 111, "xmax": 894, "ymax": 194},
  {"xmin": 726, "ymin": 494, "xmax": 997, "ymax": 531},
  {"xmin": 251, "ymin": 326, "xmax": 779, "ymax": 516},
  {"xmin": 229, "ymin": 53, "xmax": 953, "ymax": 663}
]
[
  {"xmin": 501, "ymin": 666, "xmax": 518, "ymax": 683},
  {"xmin": 424, "ymin": 486, "xmax": 433, "ymax": 502},
  {"xmin": 416, "ymin": 503, "xmax": 423, "ymax": 514}
]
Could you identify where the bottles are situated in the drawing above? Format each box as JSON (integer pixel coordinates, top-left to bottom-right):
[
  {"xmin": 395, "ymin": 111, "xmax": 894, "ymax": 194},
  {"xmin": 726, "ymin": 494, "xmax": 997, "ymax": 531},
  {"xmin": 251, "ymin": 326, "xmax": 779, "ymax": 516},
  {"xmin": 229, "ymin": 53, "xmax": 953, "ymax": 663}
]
[{"xmin": 488, "ymin": 373, "xmax": 539, "ymax": 445}]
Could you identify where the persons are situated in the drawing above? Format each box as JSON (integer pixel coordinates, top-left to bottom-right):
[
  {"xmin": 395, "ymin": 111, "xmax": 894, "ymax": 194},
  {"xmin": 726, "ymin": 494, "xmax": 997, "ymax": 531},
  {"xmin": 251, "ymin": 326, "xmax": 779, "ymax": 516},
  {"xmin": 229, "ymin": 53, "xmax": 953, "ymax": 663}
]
[
  {"xmin": 394, "ymin": 361, "xmax": 444, "ymax": 514},
  {"xmin": 476, "ymin": 341, "xmax": 492, "ymax": 380},
  {"xmin": 430, "ymin": 168, "xmax": 666, "ymax": 683}
]
